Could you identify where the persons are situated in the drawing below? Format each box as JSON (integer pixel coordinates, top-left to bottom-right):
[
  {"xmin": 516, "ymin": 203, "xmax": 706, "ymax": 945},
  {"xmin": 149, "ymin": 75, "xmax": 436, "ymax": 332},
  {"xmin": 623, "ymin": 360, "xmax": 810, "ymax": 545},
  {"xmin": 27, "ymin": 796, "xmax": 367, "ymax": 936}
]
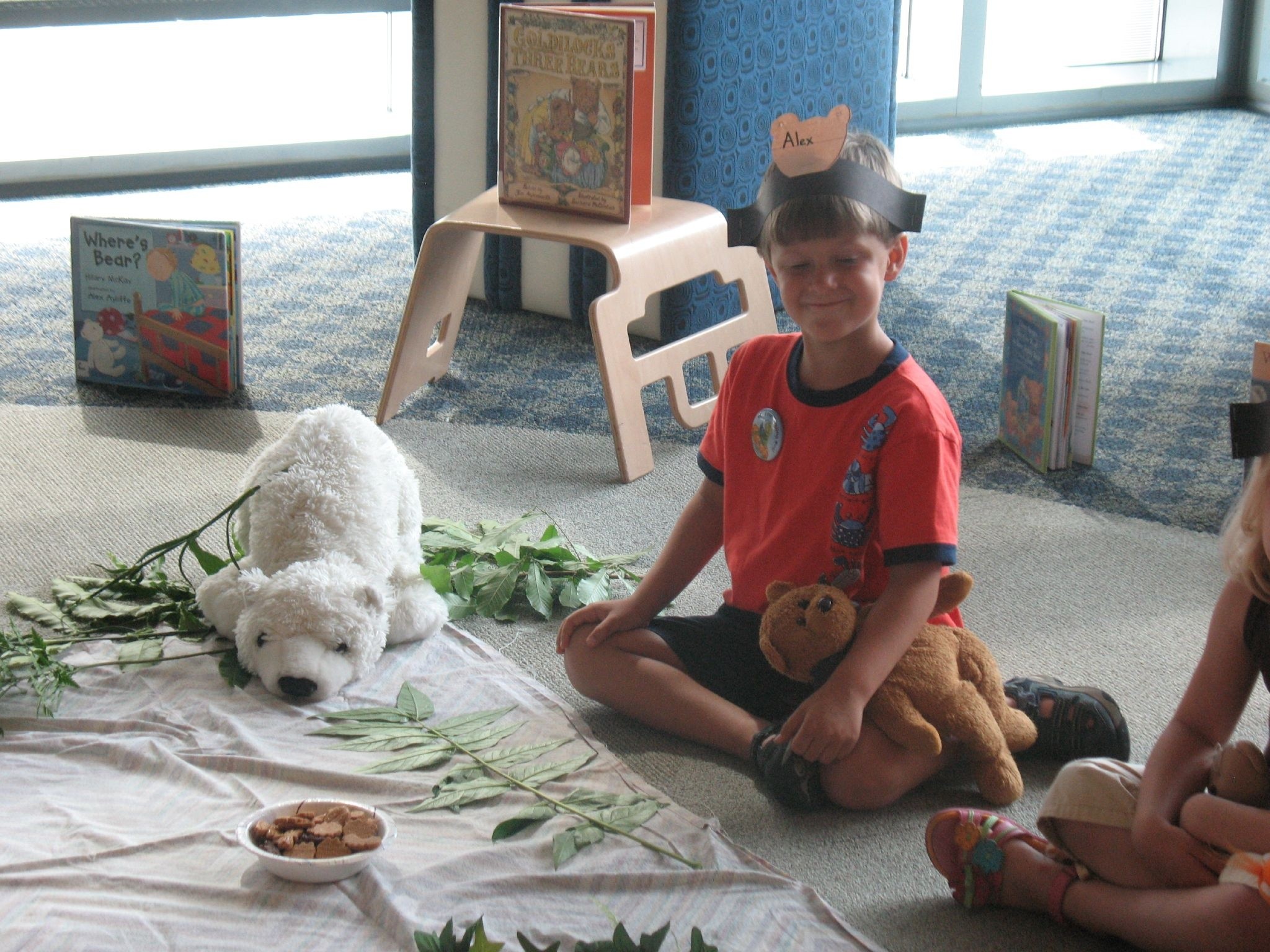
[
  {"xmin": 557, "ymin": 104, "xmax": 1130, "ymax": 814},
  {"xmin": 924, "ymin": 401, "xmax": 1270, "ymax": 952}
]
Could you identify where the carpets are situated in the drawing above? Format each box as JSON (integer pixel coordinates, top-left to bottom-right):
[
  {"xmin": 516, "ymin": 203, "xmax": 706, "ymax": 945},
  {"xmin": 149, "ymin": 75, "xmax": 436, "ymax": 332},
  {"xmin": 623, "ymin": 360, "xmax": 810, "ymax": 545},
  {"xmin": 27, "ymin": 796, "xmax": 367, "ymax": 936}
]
[{"xmin": 0, "ymin": 402, "xmax": 1270, "ymax": 952}]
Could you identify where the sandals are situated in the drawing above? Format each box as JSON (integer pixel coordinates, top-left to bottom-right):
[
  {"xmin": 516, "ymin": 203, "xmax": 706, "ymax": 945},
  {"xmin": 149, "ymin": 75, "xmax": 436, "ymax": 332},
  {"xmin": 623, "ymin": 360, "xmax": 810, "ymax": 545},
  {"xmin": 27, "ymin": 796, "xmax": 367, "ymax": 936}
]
[
  {"xmin": 925, "ymin": 808, "xmax": 1091, "ymax": 928},
  {"xmin": 749, "ymin": 721, "xmax": 827, "ymax": 810},
  {"xmin": 1003, "ymin": 674, "xmax": 1130, "ymax": 764}
]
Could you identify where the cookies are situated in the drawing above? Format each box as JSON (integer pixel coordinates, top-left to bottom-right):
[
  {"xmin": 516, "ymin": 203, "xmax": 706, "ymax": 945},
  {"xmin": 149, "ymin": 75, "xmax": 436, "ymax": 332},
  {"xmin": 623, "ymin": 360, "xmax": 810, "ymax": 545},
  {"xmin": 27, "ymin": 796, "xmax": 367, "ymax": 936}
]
[{"xmin": 254, "ymin": 807, "xmax": 381, "ymax": 860}]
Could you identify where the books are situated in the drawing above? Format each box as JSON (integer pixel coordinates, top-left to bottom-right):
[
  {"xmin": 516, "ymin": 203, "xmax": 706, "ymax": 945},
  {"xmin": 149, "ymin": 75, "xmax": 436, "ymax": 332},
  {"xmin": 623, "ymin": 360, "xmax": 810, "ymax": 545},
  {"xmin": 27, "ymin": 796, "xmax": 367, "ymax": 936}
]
[
  {"xmin": 997, "ymin": 288, "xmax": 1104, "ymax": 473},
  {"xmin": 68, "ymin": 215, "xmax": 244, "ymax": 400},
  {"xmin": 498, "ymin": 3, "xmax": 654, "ymax": 224}
]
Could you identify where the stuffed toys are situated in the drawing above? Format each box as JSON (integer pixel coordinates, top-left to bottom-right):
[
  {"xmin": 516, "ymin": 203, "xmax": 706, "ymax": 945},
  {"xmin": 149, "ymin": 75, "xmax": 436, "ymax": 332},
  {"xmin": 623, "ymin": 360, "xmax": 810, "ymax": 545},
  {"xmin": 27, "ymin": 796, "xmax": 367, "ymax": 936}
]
[
  {"xmin": 1208, "ymin": 738, "xmax": 1270, "ymax": 811},
  {"xmin": 758, "ymin": 573, "xmax": 1038, "ymax": 808},
  {"xmin": 194, "ymin": 403, "xmax": 448, "ymax": 707}
]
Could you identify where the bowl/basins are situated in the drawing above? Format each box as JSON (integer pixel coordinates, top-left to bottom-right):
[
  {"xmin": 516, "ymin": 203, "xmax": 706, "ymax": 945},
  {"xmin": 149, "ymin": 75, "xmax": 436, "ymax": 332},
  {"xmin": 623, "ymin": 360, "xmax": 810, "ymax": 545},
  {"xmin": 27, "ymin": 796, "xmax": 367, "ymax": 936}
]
[{"xmin": 236, "ymin": 798, "xmax": 398, "ymax": 882}]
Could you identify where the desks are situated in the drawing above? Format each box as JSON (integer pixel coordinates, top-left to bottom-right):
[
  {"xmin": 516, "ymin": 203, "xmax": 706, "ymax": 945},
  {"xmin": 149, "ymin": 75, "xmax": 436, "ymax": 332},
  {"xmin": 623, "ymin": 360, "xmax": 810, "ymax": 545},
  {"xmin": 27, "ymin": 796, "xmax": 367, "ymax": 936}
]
[{"xmin": 377, "ymin": 180, "xmax": 780, "ymax": 481}]
